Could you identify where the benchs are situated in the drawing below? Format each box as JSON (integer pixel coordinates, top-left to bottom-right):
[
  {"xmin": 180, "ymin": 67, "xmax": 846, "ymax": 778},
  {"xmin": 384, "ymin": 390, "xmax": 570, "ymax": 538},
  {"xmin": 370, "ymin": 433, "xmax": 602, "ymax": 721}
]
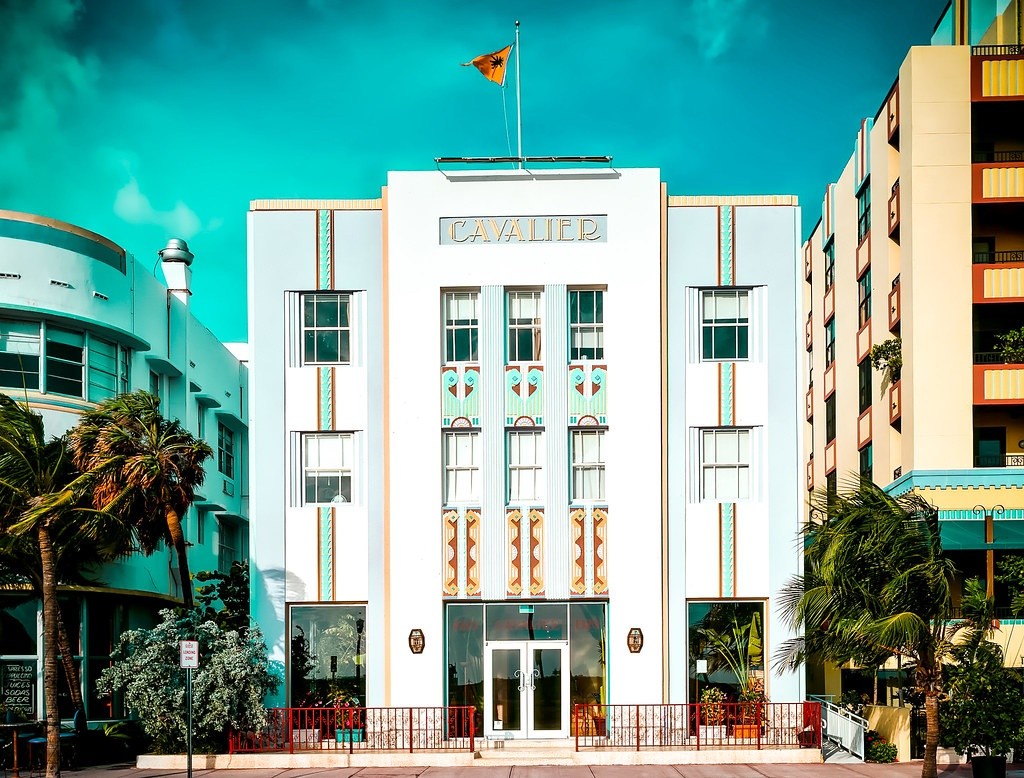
[{"xmin": 0, "ymin": 723, "xmax": 36, "ymax": 778}]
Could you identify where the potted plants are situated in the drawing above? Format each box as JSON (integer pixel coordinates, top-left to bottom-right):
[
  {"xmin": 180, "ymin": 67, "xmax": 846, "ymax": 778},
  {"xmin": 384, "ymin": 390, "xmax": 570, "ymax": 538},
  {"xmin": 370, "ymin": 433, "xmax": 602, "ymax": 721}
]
[
  {"xmin": 699, "ymin": 610, "xmax": 770, "ymax": 738},
  {"xmin": 869, "ymin": 337, "xmax": 901, "ymax": 386},
  {"xmin": 942, "ymin": 668, "xmax": 1024, "ymax": 778}
]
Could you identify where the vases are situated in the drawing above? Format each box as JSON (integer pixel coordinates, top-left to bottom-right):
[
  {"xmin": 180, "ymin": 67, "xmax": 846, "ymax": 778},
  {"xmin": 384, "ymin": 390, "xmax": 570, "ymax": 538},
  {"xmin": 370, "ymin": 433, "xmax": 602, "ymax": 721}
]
[
  {"xmin": 593, "ymin": 716, "xmax": 607, "ymax": 736},
  {"xmin": 336, "ymin": 729, "xmax": 364, "ymax": 743},
  {"xmin": 292, "ymin": 729, "xmax": 323, "ymax": 743}
]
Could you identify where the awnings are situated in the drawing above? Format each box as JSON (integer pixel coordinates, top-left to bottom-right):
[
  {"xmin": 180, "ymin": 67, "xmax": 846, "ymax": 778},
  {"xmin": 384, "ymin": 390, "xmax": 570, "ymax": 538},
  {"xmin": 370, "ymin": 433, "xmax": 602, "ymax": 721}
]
[{"xmin": 938, "ymin": 520, "xmax": 1024, "ymax": 550}]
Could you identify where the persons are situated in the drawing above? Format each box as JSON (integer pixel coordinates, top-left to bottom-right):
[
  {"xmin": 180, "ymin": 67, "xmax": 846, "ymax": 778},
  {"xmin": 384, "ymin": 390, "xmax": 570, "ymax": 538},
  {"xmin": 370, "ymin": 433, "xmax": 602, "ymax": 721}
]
[{"xmin": 841, "ymin": 689, "xmax": 873, "ymax": 723}]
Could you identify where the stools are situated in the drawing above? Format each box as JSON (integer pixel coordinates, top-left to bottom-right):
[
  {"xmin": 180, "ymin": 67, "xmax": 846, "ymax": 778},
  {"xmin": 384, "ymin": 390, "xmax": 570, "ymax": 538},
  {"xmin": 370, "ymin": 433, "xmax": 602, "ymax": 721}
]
[{"xmin": 0, "ymin": 732, "xmax": 76, "ymax": 778}]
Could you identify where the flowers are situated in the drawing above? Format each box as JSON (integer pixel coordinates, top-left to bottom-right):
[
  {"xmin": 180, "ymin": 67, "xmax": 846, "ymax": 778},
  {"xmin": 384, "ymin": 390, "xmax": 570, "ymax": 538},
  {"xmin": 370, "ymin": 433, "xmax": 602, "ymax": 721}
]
[{"xmin": 295, "ymin": 684, "xmax": 366, "ymax": 728}]
